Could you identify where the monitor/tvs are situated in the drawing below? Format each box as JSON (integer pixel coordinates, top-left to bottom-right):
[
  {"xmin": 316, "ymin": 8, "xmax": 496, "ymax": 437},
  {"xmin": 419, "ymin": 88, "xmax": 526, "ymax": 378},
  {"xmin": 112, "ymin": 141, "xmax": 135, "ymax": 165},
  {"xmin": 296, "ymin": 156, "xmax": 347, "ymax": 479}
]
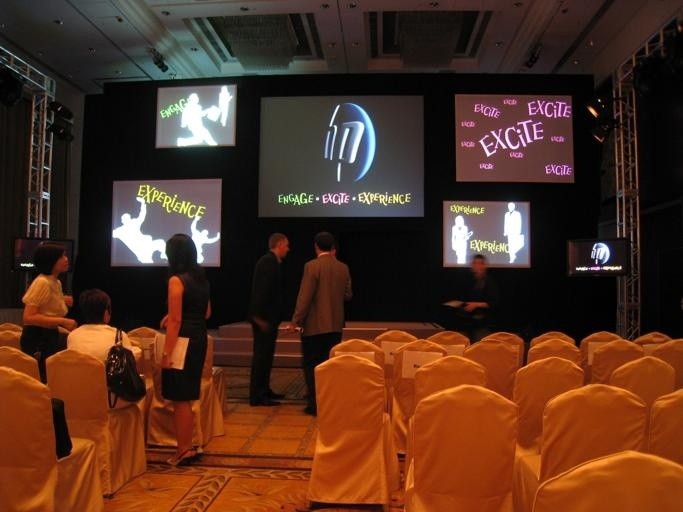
[
  {"xmin": 566, "ymin": 238, "xmax": 631, "ymax": 277},
  {"xmin": 11, "ymin": 235, "xmax": 75, "ymax": 274}
]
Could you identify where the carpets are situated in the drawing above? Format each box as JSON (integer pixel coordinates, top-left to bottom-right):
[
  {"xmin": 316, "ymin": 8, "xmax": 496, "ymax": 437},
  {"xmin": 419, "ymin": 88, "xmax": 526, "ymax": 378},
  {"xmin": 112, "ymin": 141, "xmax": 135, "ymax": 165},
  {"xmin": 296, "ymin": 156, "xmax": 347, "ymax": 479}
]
[{"xmin": 224, "ymin": 365, "xmax": 308, "ymax": 403}]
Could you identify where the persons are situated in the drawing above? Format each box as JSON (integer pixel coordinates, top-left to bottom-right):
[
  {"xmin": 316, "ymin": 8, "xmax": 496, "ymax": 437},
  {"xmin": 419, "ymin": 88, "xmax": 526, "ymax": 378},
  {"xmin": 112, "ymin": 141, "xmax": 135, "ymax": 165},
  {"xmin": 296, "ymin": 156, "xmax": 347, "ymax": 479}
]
[
  {"xmin": 218, "ymin": 85, "xmax": 232, "ymax": 127},
  {"xmin": 67, "ymin": 287, "xmax": 137, "ymax": 410},
  {"xmin": 112, "ymin": 197, "xmax": 168, "ymax": 263},
  {"xmin": 190, "ymin": 215, "xmax": 220, "ymax": 264},
  {"xmin": 160, "ymin": 233, "xmax": 213, "ymax": 466},
  {"xmin": 456, "ymin": 254, "xmax": 515, "ymax": 333},
  {"xmin": 20, "ymin": 242, "xmax": 76, "ymax": 387},
  {"xmin": 177, "ymin": 93, "xmax": 218, "ymax": 146},
  {"xmin": 451, "ymin": 214, "xmax": 473, "ymax": 264},
  {"xmin": 290, "ymin": 232, "xmax": 353, "ymax": 416},
  {"xmin": 503, "ymin": 202, "xmax": 522, "ymax": 263},
  {"xmin": 248, "ymin": 233, "xmax": 291, "ymax": 406}
]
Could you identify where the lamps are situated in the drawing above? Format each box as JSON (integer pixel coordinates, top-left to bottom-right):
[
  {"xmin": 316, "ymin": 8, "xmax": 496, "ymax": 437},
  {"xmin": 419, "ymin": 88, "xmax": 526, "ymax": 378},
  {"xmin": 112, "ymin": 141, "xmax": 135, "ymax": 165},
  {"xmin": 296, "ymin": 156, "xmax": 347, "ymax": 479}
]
[
  {"xmin": 591, "ymin": 114, "xmax": 616, "ymax": 143},
  {"xmin": 518, "ymin": 42, "xmax": 543, "ymax": 71},
  {"xmin": 46, "ymin": 100, "xmax": 74, "ymax": 121},
  {"xmin": 147, "ymin": 46, "xmax": 168, "ymax": 72},
  {"xmin": 46, "ymin": 121, "xmax": 75, "ymax": 144},
  {"xmin": 585, "ymin": 92, "xmax": 623, "ymax": 116}
]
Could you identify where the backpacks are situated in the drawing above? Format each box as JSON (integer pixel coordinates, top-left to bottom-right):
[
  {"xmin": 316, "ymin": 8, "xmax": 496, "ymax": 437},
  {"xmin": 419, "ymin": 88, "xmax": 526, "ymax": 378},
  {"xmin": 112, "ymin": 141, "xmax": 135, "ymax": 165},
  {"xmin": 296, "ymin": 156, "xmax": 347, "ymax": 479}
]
[{"xmin": 106, "ymin": 329, "xmax": 146, "ymax": 408}]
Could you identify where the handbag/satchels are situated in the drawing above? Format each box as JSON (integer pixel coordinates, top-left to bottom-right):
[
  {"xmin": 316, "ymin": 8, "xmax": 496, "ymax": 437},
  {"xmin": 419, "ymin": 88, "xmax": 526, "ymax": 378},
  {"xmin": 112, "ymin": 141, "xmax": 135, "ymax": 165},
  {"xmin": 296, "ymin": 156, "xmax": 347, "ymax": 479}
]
[{"xmin": 52, "ymin": 398, "xmax": 72, "ymax": 460}]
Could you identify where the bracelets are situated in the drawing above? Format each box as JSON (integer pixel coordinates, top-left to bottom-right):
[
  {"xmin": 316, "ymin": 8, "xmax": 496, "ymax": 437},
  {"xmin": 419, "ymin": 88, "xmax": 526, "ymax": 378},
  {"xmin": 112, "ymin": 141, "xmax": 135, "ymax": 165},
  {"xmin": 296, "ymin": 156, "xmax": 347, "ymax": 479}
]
[{"xmin": 162, "ymin": 353, "xmax": 168, "ymax": 355}]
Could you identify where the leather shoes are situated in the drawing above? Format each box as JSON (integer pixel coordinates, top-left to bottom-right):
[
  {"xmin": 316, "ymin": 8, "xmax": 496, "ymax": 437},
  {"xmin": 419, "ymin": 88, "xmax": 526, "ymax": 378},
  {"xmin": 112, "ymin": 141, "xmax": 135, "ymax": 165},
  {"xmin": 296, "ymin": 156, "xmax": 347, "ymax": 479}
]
[
  {"xmin": 303, "ymin": 395, "xmax": 316, "ymax": 415},
  {"xmin": 249, "ymin": 394, "xmax": 285, "ymax": 406},
  {"xmin": 167, "ymin": 448, "xmax": 197, "ymax": 466}
]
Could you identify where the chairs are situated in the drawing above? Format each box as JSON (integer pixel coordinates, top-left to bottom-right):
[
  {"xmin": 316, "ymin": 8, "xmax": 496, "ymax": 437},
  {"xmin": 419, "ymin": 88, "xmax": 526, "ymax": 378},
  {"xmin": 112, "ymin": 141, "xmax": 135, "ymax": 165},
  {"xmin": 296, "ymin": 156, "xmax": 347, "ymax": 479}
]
[{"xmin": 0, "ymin": 323, "xmax": 226, "ymax": 511}]
